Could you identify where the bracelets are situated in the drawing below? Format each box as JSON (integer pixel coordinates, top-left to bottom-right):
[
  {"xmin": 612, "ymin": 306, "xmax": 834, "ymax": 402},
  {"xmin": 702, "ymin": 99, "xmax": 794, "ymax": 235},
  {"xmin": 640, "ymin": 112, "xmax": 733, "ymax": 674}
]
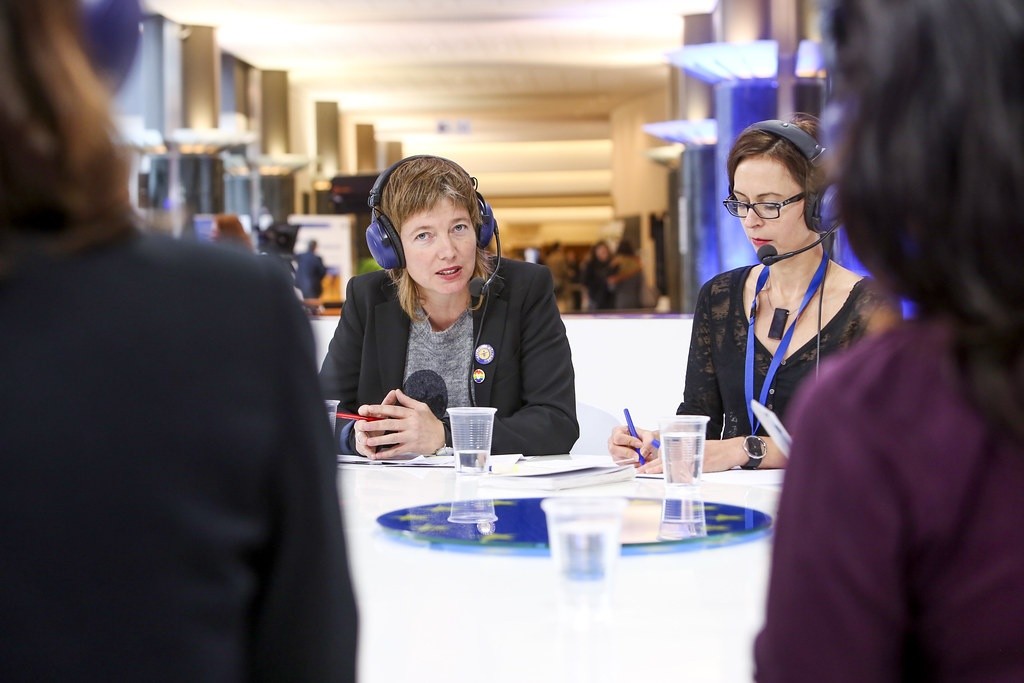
[{"xmin": 743, "ymin": 433, "xmax": 768, "ymax": 470}]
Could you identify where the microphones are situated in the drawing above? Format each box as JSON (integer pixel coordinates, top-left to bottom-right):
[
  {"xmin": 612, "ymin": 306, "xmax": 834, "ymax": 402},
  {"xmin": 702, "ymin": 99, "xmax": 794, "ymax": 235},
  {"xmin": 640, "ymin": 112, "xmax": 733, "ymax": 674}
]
[
  {"xmin": 468, "ymin": 224, "xmax": 500, "ymax": 295},
  {"xmin": 757, "ymin": 221, "xmax": 837, "ymax": 265}
]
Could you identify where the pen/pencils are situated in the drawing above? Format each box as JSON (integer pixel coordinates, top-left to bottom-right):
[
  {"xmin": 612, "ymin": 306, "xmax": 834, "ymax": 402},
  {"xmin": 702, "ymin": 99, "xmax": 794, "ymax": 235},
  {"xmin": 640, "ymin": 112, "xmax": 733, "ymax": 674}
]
[
  {"xmin": 623, "ymin": 408, "xmax": 647, "ymax": 465},
  {"xmin": 336, "ymin": 413, "xmax": 385, "ymax": 422}
]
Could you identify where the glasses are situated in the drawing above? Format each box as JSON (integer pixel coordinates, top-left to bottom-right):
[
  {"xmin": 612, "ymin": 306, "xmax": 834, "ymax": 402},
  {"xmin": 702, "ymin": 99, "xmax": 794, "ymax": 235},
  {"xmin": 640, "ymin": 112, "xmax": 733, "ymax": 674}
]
[{"xmin": 723, "ymin": 191, "xmax": 806, "ymax": 219}]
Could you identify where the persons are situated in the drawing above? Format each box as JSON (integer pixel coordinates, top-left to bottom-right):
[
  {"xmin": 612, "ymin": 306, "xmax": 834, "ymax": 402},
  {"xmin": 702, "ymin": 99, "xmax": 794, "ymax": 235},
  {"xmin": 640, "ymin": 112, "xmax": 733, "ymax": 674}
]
[
  {"xmin": 296, "ymin": 242, "xmax": 328, "ymax": 312},
  {"xmin": 315, "ymin": 157, "xmax": 583, "ymax": 463},
  {"xmin": 504, "ymin": 237, "xmax": 646, "ymax": 311},
  {"xmin": 754, "ymin": 0, "xmax": 1024, "ymax": 683},
  {"xmin": 0, "ymin": 0, "xmax": 363, "ymax": 683},
  {"xmin": 608, "ymin": 115, "xmax": 898, "ymax": 478},
  {"xmin": 211, "ymin": 214, "xmax": 255, "ymax": 252}
]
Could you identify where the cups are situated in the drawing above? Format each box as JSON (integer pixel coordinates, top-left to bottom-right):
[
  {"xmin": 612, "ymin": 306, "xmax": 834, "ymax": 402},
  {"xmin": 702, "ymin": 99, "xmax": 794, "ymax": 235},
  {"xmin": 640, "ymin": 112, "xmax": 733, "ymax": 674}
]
[
  {"xmin": 540, "ymin": 496, "xmax": 625, "ymax": 594},
  {"xmin": 658, "ymin": 415, "xmax": 711, "ymax": 489},
  {"xmin": 657, "ymin": 494, "xmax": 708, "ymax": 539},
  {"xmin": 323, "ymin": 400, "xmax": 342, "ymax": 436},
  {"xmin": 446, "ymin": 407, "xmax": 498, "ymax": 472},
  {"xmin": 448, "ymin": 475, "xmax": 499, "ymax": 523}
]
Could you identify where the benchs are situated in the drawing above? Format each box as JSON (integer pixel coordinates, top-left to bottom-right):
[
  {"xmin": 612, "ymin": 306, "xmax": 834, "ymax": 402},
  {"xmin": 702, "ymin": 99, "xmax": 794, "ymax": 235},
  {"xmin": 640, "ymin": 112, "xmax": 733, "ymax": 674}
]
[{"xmin": 309, "ymin": 313, "xmax": 692, "ymax": 452}]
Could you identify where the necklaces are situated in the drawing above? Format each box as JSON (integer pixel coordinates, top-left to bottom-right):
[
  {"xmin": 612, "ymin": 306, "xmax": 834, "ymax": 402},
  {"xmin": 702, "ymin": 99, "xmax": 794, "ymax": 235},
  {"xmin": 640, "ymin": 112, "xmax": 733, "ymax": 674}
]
[{"xmin": 765, "ymin": 280, "xmax": 820, "ymax": 340}]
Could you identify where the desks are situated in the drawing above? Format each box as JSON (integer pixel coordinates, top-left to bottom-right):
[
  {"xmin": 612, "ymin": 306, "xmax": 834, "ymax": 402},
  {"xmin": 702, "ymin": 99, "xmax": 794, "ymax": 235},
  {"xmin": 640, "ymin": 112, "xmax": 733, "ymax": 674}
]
[{"xmin": 332, "ymin": 452, "xmax": 786, "ymax": 683}]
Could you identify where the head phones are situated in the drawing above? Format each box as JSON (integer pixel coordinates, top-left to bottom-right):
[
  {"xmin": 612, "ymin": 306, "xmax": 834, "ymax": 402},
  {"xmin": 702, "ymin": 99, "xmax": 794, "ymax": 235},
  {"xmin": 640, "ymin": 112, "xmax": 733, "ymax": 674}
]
[
  {"xmin": 736, "ymin": 119, "xmax": 845, "ymax": 233},
  {"xmin": 364, "ymin": 155, "xmax": 495, "ymax": 270},
  {"xmin": 78, "ymin": 0, "xmax": 143, "ymax": 98}
]
